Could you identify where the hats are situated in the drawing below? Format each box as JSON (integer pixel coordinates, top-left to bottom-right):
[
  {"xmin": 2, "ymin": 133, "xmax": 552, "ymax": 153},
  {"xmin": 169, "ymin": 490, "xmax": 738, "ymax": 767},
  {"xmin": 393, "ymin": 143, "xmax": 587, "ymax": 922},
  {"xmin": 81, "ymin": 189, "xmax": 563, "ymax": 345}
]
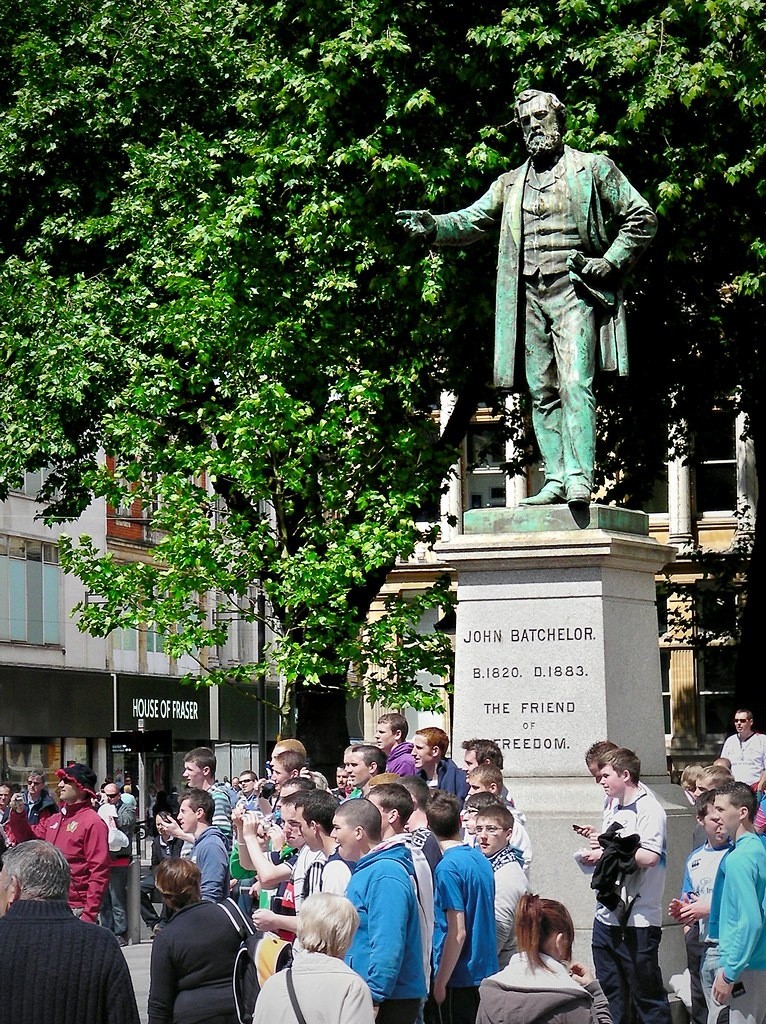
[{"xmin": 55, "ymin": 763, "xmax": 98, "ymax": 800}]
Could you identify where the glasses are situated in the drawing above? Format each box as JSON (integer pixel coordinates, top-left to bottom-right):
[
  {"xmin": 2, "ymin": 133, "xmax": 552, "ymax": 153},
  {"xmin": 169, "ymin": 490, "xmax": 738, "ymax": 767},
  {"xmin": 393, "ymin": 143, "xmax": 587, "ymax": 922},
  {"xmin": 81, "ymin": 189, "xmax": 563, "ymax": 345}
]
[
  {"xmin": 125, "ymin": 780, "xmax": 131, "ymax": 783},
  {"xmin": 239, "ymin": 779, "xmax": 253, "ymax": 784},
  {"xmin": 466, "ymin": 810, "xmax": 478, "ymax": 814},
  {"xmin": 106, "ymin": 793, "xmax": 119, "ymax": 798},
  {"xmin": 59, "ymin": 777, "xmax": 71, "ymax": 784},
  {"xmin": 26, "ymin": 779, "xmax": 41, "ymax": 785},
  {"xmin": 474, "ymin": 825, "xmax": 504, "ymax": 836},
  {"xmin": 733, "ymin": 719, "xmax": 746, "ymax": 723}
]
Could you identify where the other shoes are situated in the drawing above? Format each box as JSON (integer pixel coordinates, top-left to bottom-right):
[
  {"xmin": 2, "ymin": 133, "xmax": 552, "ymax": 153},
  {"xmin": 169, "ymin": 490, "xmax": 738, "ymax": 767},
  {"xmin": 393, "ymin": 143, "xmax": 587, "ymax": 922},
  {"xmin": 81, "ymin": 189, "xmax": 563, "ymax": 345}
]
[
  {"xmin": 150, "ymin": 922, "xmax": 160, "ymax": 938},
  {"xmin": 116, "ymin": 934, "xmax": 128, "ymax": 948}
]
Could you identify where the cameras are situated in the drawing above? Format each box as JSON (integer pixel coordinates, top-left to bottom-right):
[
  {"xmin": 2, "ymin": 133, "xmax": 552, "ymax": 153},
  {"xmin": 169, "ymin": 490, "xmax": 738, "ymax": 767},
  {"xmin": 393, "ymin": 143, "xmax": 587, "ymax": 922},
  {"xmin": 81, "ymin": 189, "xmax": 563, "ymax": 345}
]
[{"xmin": 16, "ymin": 793, "xmax": 26, "ymax": 802}]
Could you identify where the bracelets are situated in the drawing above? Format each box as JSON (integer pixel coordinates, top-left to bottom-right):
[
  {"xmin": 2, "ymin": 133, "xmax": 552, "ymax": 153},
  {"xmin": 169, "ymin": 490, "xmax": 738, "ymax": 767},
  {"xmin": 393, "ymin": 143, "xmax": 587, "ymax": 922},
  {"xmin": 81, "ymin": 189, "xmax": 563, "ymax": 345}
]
[{"xmin": 237, "ymin": 843, "xmax": 245, "ymax": 846}]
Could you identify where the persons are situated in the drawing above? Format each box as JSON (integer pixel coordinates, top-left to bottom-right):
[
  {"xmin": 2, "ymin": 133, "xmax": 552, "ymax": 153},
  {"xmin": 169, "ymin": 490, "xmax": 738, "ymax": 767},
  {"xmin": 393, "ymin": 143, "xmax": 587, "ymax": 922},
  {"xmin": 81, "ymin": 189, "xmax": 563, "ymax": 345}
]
[
  {"xmin": 476, "ymin": 893, "xmax": 613, "ymax": 1024},
  {"xmin": 667, "ymin": 709, "xmax": 766, "ymax": 1024},
  {"xmin": 395, "ymin": 90, "xmax": 657, "ymax": 505},
  {"xmin": 572, "ymin": 740, "xmax": 674, "ymax": 1024},
  {"xmin": 0, "ymin": 713, "xmax": 533, "ymax": 1024}
]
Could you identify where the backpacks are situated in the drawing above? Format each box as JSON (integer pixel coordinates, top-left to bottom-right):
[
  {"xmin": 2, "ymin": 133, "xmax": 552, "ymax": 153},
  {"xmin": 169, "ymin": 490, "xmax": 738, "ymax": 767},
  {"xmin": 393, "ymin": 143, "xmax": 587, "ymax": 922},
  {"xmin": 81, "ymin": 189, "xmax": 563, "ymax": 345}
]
[{"xmin": 218, "ymin": 896, "xmax": 294, "ymax": 1024}]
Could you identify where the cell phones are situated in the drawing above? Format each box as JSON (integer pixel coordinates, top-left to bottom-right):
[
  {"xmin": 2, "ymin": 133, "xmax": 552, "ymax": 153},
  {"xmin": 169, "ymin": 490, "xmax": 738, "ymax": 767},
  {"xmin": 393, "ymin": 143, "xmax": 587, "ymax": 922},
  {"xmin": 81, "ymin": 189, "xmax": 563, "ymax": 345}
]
[
  {"xmin": 573, "ymin": 825, "xmax": 585, "ymax": 831},
  {"xmin": 159, "ymin": 812, "xmax": 172, "ymax": 823}
]
